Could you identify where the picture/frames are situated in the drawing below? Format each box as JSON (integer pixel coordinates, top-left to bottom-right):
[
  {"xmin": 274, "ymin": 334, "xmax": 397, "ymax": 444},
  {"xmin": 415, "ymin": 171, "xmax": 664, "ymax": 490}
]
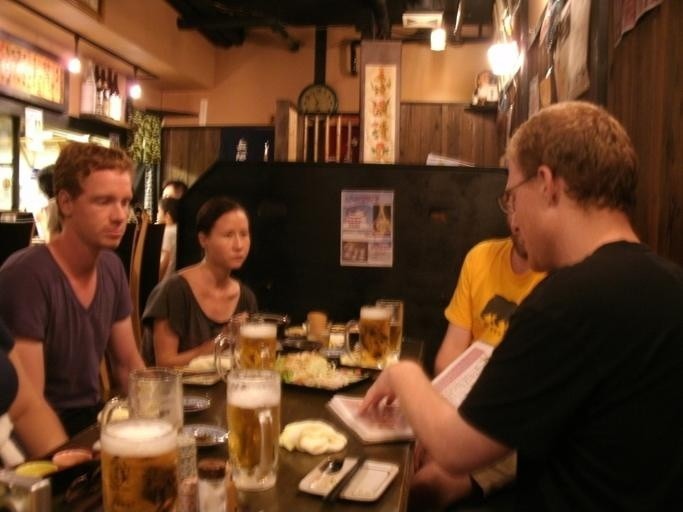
[{"xmin": 0, "ymin": 30, "xmax": 69, "ymax": 116}]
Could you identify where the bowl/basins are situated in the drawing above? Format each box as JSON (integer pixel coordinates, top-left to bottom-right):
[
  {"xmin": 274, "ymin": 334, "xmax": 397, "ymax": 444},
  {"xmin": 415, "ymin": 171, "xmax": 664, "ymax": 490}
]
[{"xmin": 282, "ymin": 338, "xmax": 322, "ymax": 357}]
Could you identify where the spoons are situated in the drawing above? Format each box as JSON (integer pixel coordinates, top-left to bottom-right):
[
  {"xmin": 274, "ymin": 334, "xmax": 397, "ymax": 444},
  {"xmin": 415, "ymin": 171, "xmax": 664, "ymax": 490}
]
[{"xmin": 308, "ymin": 454, "xmax": 346, "ymax": 490}]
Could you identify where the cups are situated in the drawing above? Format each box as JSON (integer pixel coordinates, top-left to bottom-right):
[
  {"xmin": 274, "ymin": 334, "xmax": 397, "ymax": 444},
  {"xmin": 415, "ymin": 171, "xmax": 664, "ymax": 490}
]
[
  {"xmin": 225, "ymin": 367, "xmax": 283, "ymax": 493},
  {"xmin": 303, "ymin": 319, "xmax": 334, "ymax": 351},
  {"xmin": 210, "ymin": 320, "xmax": 238, "ymax": 385},
  {"xmin": 374, "ymin": 299, "xmax": 405, "ymax": 362},
  {"xmin": 171, "ymin": 430, "xmax": 202, "ymax": 512},
  {"xmin": 98, "ymin": 366, "xmax": 185, "ymax": 433},
  {"xmin": 237, "ymin": 323, "xmax": 279, "ymax": 373},
  {"xmin": 100, "ymin": 417, "xmax": 181, "ymax": 512},
  {"xmin": 343, "ymin": 305, "xmax": 392, "ymax": 371}
]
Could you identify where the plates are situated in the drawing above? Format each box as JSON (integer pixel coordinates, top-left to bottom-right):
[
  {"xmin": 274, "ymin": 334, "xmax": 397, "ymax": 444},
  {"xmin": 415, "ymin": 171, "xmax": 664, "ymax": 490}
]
[
  {"xmin": 297, "ymin": 457, "xmax": 400, "ymax": 503},
  {"xmin": 180, "ymin": 421, "xmax": 229, "ymax": 449},
  {"xmin": 182, "ymin": 394, "xmax": 213, "ymax": 412}
]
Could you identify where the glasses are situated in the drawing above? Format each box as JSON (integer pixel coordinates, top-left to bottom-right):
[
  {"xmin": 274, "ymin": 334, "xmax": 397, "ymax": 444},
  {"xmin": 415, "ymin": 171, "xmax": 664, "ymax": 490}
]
[{"xmin": 496, "ymin": 166, "xmax": 547, "ymax": 216}]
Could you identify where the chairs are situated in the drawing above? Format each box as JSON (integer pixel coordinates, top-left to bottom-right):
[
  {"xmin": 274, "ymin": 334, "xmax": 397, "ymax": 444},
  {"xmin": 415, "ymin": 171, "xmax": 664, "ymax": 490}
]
[
  {"xmin": 0, "ymin": 218, "xmax": 35, "ymax": 270},
  {"xmin": 98, "ymin": 210, "xmax": 151, "ymax": 403},
  {"xmin": 130, "ymin": 220, "xmax": 168, "ymax": 354}
]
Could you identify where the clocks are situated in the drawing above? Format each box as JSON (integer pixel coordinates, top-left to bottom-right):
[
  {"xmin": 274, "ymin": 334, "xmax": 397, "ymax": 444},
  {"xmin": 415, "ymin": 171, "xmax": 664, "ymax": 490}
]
[{"xmin": 297, "ymin": 82, "xmax": 337, "ymax": 124}]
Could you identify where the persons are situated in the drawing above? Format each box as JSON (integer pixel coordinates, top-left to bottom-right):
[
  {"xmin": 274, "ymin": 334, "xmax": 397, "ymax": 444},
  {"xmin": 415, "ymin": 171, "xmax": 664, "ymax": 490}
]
[
  {"xmin": 161, "ymin": 182, "xmax": 185, "ymax": 198},
  {"xmin": 413, "ymin": 218, "xmax": 549, "ymax": 496},
  {"xmin": 143, "ymin": 198, "xmax": 256, "ymax": 368},
  {"xmin": 158, "ymin": 200, "xmax": 179, "ymax": 283},
  {"xmin": 0, "ymin": 142, "xmax": 150, "ymax": 461},
  {"xmin": 352, "ymin": 101, "xmax": 677, "ymax": 512}
]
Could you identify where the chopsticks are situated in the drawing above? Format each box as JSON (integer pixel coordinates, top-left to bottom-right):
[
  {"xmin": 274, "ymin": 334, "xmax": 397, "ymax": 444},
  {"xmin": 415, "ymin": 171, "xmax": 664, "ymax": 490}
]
[{"xmin": 325, "ymin": 457, "xmax": 365, "ymax": 505}]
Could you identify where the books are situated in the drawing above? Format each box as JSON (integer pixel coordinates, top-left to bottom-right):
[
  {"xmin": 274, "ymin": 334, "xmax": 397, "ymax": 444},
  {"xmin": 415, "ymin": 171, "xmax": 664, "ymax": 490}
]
[{"xmin": 329, "ymin": 341, "xmax": 495, "ymax": 446}]
